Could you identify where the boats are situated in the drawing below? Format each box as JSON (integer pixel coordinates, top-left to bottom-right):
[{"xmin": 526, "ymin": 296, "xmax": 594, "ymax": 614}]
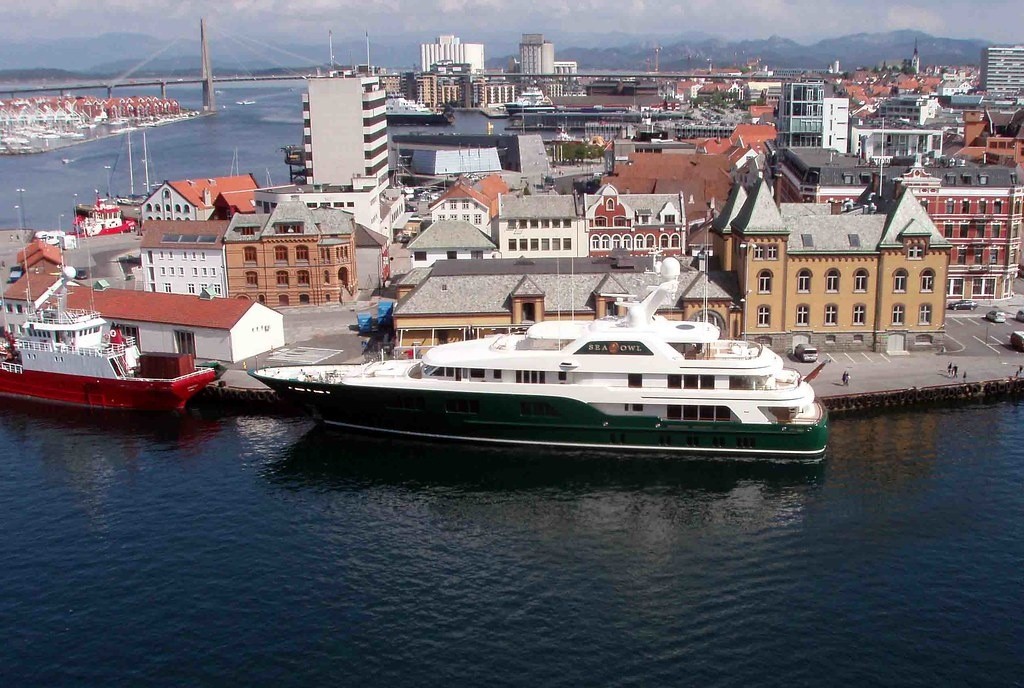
[
  {"xmin": 502, "ymin": 84, "xmax": 562, "ymax": 117},
  {"xmin": 385, "ymin": 95, "xmax": 455, "ymax": 126},
  {"xmin": 0, "ymin": 204, "xmax": 216, "ymax": 414},
  {"xmin": 246, "ymin": 216, "xmax": 829, "ymax": 459},
  {"xmin": 60, "ymin": 186, "xmax": 137, "ymax": 239}
]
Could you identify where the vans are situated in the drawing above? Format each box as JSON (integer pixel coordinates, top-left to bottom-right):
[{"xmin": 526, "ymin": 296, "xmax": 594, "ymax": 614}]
[
  {"xmin": 794, "ymin": 344, "xmax": 818, "ymax": 362},
  {"xmin": 1011, "ymin": 330, "xmax": 1024, "ymax": 351}
]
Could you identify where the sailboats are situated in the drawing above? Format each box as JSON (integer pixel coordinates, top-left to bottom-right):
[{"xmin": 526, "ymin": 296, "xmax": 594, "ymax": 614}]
[{"xmin": 104, "ymin": 118, "xmax": 158, "ymax": 221}]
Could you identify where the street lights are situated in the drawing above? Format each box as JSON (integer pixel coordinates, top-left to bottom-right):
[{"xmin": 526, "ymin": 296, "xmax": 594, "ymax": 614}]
[
  {"xmin": 739, "ymin": 243, "xmax": 757, "ymax": 340},
  {"xmin": 15, "ymin": 188, "xmax": 27, "ymax": 241}
]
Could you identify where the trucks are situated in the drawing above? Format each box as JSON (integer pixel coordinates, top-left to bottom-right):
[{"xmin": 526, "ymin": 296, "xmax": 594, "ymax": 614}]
[
  {"xmin": 357, "ymin": 314, "xmax": 372, "ymax": 333},
  {"xmin": 378, "ymin": 301, "xmax": 393, "ymax": 329}
]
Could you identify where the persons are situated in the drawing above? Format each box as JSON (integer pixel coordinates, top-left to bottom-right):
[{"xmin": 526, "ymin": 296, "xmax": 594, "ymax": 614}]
[
  {"xmin": 1016, "ymin": 365, "xmax": 1023, "ymax": 377},
  {"xmin": 947, "ymin": 362, "xmax": 967, "ymax": 383},
  {"xmin": 2, "ymin": 347, "xmax": 19, "ymax": 362},
  {"xmin": 842, "ymin": 371, "xmax": 850, "ymax": 386},
  {"xmin": 304, "ymin": 375, "xmax": 313, "ymax": 382}
]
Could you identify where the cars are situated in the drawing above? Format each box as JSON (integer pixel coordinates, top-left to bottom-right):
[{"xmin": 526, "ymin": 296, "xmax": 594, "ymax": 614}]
[
  {"xmin": 949, "ymin": 300, "xmax": 978, "ymax": 310},
  {"xmin": 987, "ymin": 311, "xmax": 1006, "ymax": 323},
  {"xmin": 1016, "ymin": 309, "xmax": 1024, "ymax": 322}
]
